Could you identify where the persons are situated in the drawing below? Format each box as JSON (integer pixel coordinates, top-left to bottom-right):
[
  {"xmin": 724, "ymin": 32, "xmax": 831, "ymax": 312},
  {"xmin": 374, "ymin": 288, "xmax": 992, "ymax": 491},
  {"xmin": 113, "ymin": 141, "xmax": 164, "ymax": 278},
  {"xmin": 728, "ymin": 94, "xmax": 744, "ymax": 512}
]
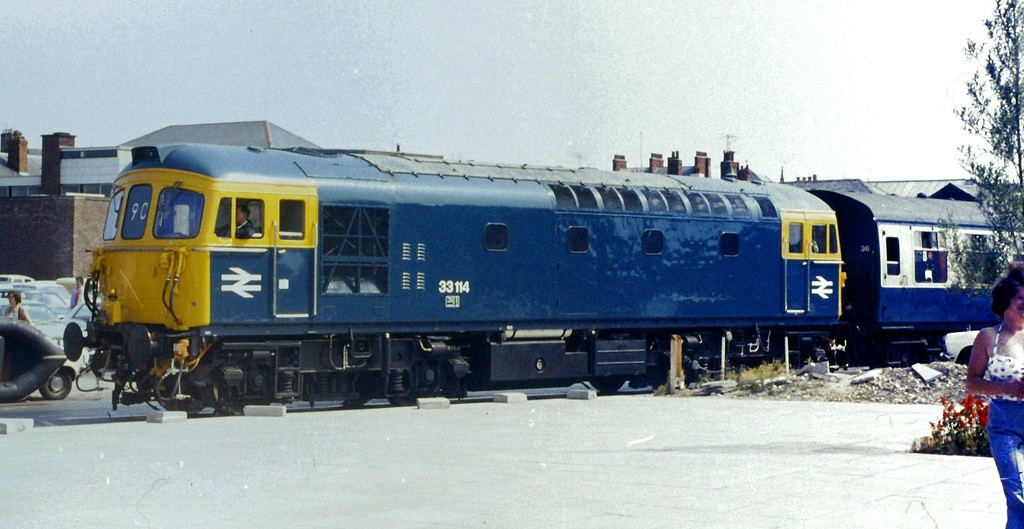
[
  {"xmin": 3, "ymin": 291, "xmax": 31, "ymax": 324},
  {"xmin": 216, "ymin": 206, "xmax": 255, "ymax": 239},
  {"xmin": 967, "ymin": 278, "xmax": 1024, "ymax": 528},
  {"xmin": 70, "ymin": 276, "xmax": 85, "ymax": 309}
]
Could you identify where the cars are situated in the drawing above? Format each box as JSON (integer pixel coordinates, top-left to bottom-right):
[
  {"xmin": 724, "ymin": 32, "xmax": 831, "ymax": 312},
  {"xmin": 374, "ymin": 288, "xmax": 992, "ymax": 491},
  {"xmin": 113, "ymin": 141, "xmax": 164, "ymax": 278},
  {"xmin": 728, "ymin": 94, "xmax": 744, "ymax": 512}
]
[{"xmin": 0, "ymin": 273, "xmax": 119, "ymax": 401}]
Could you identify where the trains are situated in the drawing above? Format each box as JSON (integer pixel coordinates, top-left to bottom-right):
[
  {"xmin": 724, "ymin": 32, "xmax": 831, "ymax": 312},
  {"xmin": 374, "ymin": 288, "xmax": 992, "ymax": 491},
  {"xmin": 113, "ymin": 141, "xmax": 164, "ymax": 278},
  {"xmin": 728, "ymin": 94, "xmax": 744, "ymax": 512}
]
[{"xmin": 65, "ymin": 142, "xmax": 1019, "ymax": 413}]
